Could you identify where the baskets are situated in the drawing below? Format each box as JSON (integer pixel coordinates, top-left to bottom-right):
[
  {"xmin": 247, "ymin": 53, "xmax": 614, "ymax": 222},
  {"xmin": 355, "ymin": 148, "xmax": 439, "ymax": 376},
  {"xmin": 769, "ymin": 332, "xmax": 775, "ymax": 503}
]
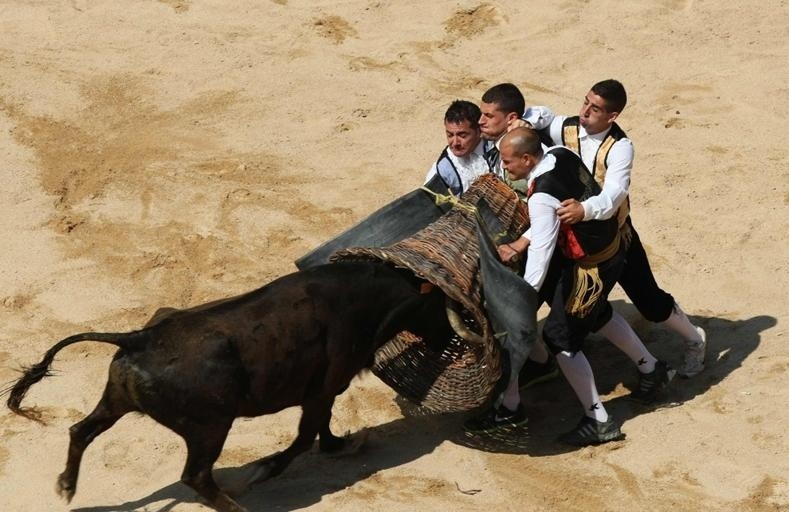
[{"xmin": 330, "ymin": 172, "xmax": 529, "ymax": 414}]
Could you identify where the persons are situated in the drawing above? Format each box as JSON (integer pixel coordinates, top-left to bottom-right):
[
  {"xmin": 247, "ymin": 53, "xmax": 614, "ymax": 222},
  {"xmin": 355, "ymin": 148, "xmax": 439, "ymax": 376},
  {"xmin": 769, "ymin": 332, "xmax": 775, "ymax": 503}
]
[
  {"xmin": 537, "ymin": 79, "xmax": 708, "ymax": 380},
  {"xmin": 497, "ymin": 124, "xmax": 671, "ymax": 446},
  {"xmin": 423, "ymin": 98, "xmax": 555, "ymax": 197},
  {"xmin": 458, "ymin": 81, "xmax": 562, "ymax": 446}
]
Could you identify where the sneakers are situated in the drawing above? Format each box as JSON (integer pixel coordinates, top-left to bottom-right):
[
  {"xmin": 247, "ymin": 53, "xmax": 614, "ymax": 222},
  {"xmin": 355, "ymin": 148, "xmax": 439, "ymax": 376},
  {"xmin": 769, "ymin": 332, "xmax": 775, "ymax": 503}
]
[
  {"xmin": 678, "ymin": 325, "xmax": 707, "ymax": 379},
  {"xmin": 519, "ymin": 352, "xmax": 561, "ymax": 390},
  {"xmin": 460, "ymin": 402, "xmax": 529, "ymax": 433},
  {"xmin": 630, "ymin": 358, "xmax": 676, "ymax": 404},
  {"xmin": 557, "ymin": 414, "xmax": 621, "ymax": 447}
]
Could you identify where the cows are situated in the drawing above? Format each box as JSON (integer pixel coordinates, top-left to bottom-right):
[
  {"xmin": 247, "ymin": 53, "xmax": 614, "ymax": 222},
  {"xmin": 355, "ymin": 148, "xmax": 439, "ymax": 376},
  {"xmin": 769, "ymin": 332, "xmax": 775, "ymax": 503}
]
[{"xmin": 5, "ymin": 254, "xmax": 512, "ymax": 511}]
